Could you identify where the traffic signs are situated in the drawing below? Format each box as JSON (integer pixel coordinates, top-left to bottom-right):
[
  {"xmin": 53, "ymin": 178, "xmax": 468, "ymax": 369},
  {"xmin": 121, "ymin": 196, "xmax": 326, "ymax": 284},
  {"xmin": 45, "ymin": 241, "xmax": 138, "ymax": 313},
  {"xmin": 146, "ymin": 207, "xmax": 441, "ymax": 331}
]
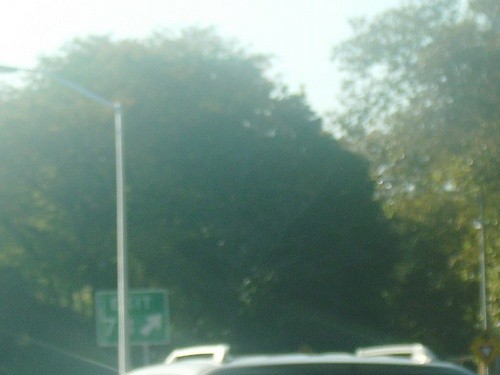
[{"xmin": 95, "ymin": 288, "xmax": 171, "ymax": 348}]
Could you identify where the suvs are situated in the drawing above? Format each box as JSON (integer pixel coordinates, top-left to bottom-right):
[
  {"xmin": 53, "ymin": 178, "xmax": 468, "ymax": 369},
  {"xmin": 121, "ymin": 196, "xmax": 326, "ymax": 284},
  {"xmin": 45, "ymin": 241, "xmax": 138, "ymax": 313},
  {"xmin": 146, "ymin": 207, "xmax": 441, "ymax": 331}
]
[{"xmin": 107, "ymin": 344, "xmax": 471, "ymax": 375}]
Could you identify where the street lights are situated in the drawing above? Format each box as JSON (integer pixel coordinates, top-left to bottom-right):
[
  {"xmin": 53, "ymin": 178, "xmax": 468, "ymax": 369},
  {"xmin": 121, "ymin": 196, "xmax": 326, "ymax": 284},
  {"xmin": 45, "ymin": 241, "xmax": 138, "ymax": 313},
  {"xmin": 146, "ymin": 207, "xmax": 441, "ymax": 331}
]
[{"xmin": 0, "ymin": 65, "xmax": 132, "ymax": 375}]
